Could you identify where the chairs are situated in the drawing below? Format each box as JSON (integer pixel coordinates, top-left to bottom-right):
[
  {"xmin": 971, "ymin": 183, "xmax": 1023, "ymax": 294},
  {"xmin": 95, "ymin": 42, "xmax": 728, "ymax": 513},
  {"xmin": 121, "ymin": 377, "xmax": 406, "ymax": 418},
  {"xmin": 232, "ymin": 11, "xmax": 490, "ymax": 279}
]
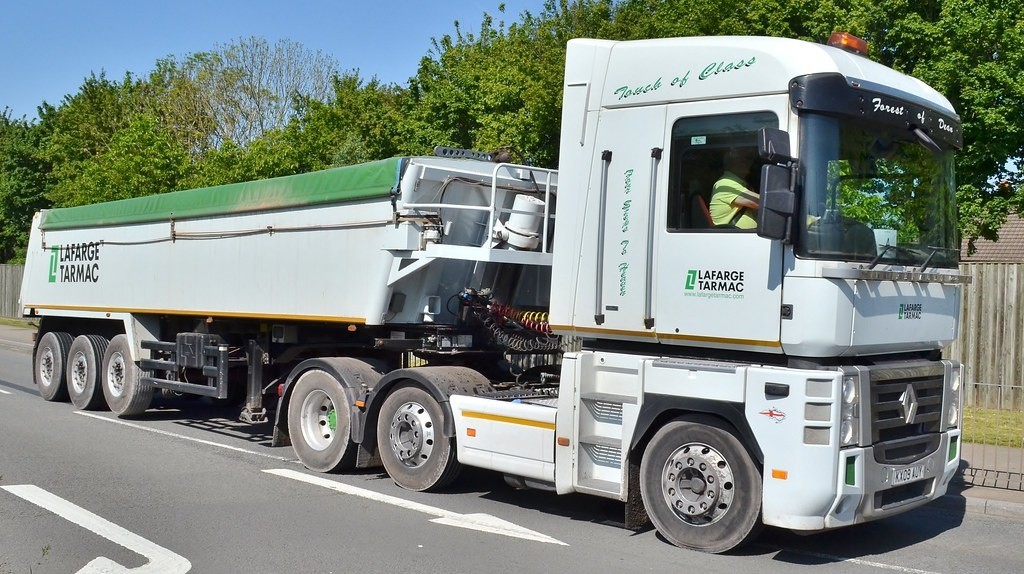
[{"xmin": 690, "ymin": 191, "xmax": 715, "ymax": 228}]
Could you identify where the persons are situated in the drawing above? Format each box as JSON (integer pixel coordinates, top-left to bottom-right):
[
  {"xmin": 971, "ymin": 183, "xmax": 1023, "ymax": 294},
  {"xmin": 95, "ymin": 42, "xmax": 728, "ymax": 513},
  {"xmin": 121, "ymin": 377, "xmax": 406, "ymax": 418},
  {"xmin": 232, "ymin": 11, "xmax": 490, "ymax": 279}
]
[{"xmin": 708, "ymin": 146, "xmax": 761, "ymax": 230}]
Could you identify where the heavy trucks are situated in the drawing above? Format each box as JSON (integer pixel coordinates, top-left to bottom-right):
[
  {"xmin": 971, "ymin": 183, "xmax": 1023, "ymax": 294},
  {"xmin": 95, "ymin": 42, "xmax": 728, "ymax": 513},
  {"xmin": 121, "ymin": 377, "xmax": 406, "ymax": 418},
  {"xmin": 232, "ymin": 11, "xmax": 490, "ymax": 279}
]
[{"xmin": 16, "ymin": 34, "xmax": 968, "ymax": 555}]
